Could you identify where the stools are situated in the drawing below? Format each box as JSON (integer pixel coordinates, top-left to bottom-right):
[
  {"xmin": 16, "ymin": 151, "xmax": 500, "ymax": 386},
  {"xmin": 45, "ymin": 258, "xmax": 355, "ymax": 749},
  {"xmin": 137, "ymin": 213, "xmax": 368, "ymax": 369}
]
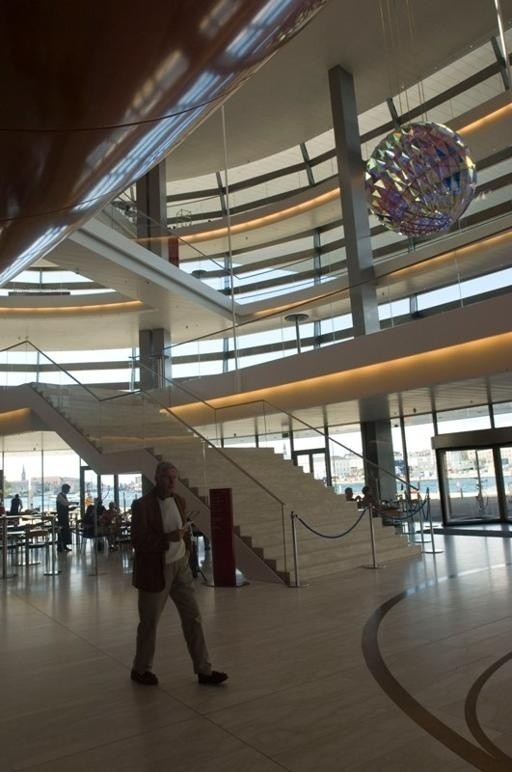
[
  {"xmin": 349, "ymin": 498, "xmax": 424, "ymax": 537},
  {"xmin": 0, "ymin": 507, "xmax": 129, "ymax": 579}
]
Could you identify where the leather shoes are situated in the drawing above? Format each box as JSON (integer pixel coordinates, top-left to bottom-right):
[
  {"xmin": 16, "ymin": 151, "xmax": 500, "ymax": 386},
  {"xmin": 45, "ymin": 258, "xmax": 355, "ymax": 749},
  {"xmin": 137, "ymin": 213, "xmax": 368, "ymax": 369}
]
[
  {"xmin": 197, "ymin": 670, "xmax": 228, "ymax": 685},
  {"xmin": 130, "ymin": 667, "xmax": 159, "ymax": 686}
]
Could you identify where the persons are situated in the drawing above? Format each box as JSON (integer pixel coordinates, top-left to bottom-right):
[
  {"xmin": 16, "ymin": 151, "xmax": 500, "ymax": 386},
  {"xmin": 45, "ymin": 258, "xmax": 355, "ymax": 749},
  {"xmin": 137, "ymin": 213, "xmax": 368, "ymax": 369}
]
[
  {"xmin": 100, "ymin": 501, "xmax": 123, "ymax": 552},
  {"xmin": 129, "ymin": 461, "xmax": 228, "ymax": 686},
  {"xmin": 10, "ymin": 494, "xmax": 23, "ymax": 515},
  {"xmin": 344, "ymin": 487, "xmax": 359, "ymax": 503},
  {"xmin": 358, "ymin": 485, "xmax": 377, "ymax": 516},
  {"xmin": 55, "ymin": 484, "xmax": 80, "ymax": 552},
  {"xmin": 84, "ymin": 498, "xmax": 107, "ymax": 551}
]
